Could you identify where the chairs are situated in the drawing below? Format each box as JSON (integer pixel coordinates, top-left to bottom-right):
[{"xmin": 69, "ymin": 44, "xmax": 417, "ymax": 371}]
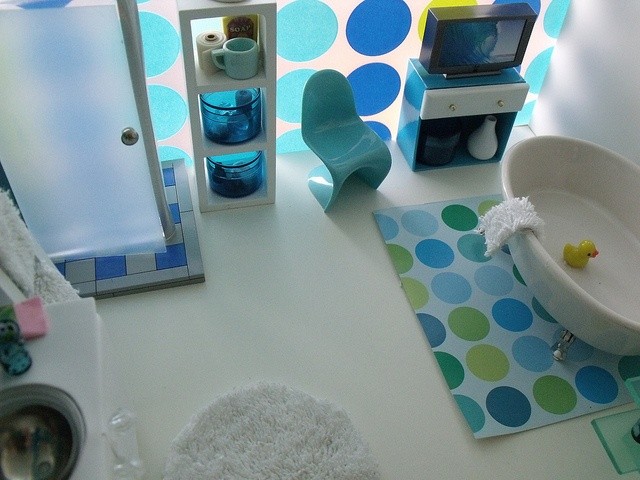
[{"xmin": 300, "ymin": 69, "xmax": 392, "ymax": 214}]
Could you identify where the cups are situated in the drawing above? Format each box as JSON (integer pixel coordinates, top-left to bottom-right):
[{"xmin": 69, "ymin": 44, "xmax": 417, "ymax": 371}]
[
  {"xmin": 212, "ymin": 37, "xmax": 258, "ymax": 79},
  {"xmin": 0, "ymin": 382, "xmax": 86, "ymax": 480}
]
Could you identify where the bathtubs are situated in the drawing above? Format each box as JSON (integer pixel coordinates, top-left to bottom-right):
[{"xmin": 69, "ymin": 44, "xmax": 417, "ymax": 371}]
[{"xmin": 498, "ymin": 132, "xmax": 640, "ymax": 363}]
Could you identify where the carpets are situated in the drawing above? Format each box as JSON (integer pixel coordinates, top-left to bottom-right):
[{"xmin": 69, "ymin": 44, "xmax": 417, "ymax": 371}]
[{"xmin": 162, "ymin": 383, "xmax": 381, "ymax": 478}]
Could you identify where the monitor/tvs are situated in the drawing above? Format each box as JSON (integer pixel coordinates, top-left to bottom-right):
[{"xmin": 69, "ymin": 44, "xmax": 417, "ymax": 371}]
[{"xmin": 418, "ymin": 3, "xmax": 537, "ymax": 79}]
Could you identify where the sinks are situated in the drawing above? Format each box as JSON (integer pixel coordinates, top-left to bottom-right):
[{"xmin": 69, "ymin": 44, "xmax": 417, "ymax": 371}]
[{"xmin": 1, "ymin": 382, "xmax": 88, "ymax": 479}]
